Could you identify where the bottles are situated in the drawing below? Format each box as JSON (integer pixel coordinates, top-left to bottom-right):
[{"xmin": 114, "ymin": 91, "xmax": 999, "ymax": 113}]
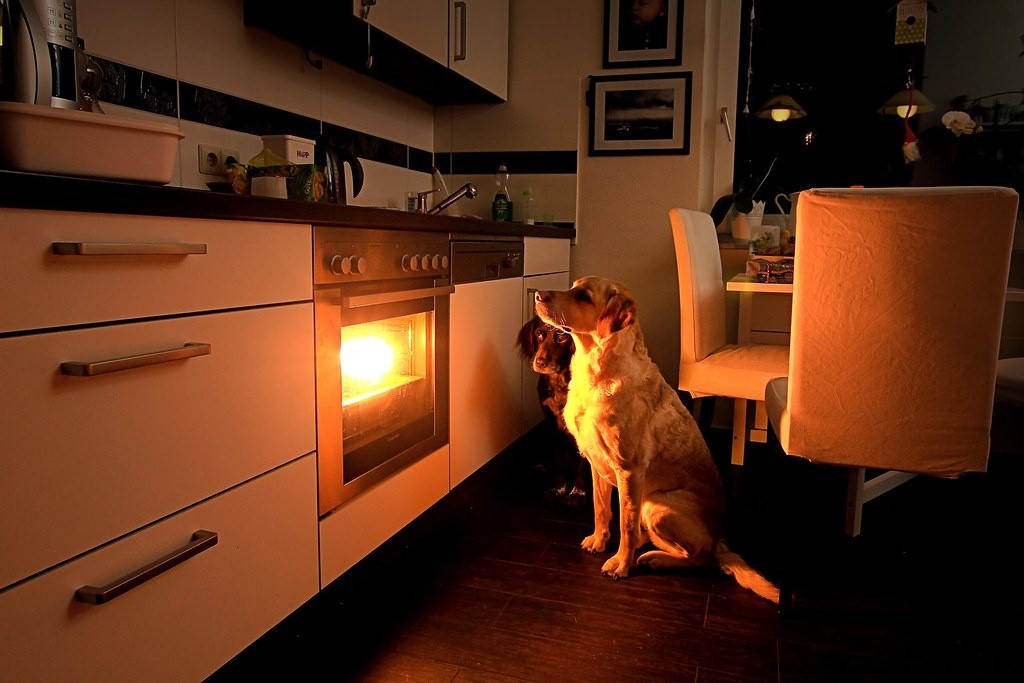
[
  {"xmin": 492, "ymin": 166, "xmax": 514, "ymax": 223},
  {"xmin": 520, "ymin": 190, "xmax": 535, "ymax": 224},
  {"xmin": 78, "ymin": 35, "xmax": 102, "ymax": 114}
]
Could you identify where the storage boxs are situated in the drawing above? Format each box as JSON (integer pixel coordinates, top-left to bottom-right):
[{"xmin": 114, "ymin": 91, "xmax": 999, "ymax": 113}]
[
  {"xmin": 0, "ymin": 100, "xmax": 186, "ymax": 182},
  {"xmin": 261, "ymin": 134, "xmax": 316, "ymax": 165}
]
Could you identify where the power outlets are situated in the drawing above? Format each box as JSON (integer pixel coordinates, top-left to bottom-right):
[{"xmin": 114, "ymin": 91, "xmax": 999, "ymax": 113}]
[
  {"xmin": 198, "ymin": 144, "xmax": 222, "ymax": 176},
  {"xmin": 220, "ymin": 147, "xmax": 239, "ymax": 174}
]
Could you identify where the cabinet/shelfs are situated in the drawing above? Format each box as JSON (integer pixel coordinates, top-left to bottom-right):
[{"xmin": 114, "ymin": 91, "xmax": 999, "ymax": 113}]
[
  {"xmin": 0, "ymin": 170, "xmax": 315, "ymax": 682},
  {"xmin": 242, "ymin": 0, "xmax": 511, "ymax": 106},
  {"xmin": 523, "ymin": 234, "xmax": 570, "ymax": 433}
]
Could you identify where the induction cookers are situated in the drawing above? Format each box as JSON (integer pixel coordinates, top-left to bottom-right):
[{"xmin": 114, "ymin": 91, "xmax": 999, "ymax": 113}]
[{"xmin": 313, "ymin": 211, "xmax": 450, "ymax": 285}]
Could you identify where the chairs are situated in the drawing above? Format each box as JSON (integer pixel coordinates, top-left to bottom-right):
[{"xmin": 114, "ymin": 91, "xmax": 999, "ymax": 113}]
[
  {"xmin": 667, "ymin": 204, "xmax": 790, "ymax": 438},
  {"xmin": 766, "ymin": 185, "xmax": 1021, "ymax": 475}
]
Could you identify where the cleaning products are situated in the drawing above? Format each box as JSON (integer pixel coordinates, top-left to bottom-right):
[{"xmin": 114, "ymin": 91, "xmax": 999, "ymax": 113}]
[
  {"xmin": 521, "ymin": 187, "xmax": 536, "ymax": 226},
  {"xmin": 491, "ymin": 165, "xmax": 512, "ymax": 221}
]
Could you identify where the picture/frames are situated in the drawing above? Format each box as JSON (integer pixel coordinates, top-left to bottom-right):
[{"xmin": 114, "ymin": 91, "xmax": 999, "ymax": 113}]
[
  {"xmin": 602, "ymin": 1, "xmax": 682, "ymax": 70},
  {"xmin": 590, "ymin": 70, "xmax": 693, "ymax": 157}
]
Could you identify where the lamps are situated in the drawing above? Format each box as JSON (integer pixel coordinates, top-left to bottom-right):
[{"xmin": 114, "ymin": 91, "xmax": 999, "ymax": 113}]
[
  {"xmin": 874, "ymin": 0, "xmax": 938, "ymax": 160},
  {"xmin": 750, "ymin": 92, "xmax": 809, "ymax": 122}
]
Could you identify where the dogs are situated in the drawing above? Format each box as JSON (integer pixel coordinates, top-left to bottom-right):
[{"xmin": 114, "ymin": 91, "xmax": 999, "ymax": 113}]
[{"xmin": 512, "ymin": 275, "xmax": 782, "ymax": 605}]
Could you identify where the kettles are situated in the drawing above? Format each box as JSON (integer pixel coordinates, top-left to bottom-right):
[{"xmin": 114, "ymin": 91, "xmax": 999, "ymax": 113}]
[{"xmin": 314, "ymin": 134, "xmax": 364, "ymax": 204}]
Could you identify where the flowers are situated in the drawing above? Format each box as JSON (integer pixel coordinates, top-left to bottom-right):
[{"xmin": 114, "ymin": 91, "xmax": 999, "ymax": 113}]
[{"xmin": 941, "ymin": 109, "xmax": 976, "ymax": 137}]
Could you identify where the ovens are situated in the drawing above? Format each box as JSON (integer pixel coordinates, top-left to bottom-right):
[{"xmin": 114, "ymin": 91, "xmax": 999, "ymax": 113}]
[{"xmin": 315, "ymin": 283, "xmax": 450, "ymax": 517}]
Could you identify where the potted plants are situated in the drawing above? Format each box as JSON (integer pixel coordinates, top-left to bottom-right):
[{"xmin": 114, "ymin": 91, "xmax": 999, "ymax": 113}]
[{"xmin": 708, "ymin": 156, "xmax": 779, "ymax": 249}]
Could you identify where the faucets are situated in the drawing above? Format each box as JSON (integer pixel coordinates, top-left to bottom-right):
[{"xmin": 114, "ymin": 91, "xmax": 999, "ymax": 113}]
[{"xmin": 417, "ymin": 182, "xmax": 477, "ymax": 215}]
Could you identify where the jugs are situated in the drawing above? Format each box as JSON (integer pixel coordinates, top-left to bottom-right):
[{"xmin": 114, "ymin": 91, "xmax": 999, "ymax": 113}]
[{"xmin": 775, "ymin": 192, "xmax": 800, "ymax": 236}]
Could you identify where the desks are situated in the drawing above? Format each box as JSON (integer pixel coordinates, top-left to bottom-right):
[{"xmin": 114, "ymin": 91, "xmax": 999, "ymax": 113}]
[{"xmin": 720, "ymin": 271, "xmax": 1024, "ymax": 535}]
